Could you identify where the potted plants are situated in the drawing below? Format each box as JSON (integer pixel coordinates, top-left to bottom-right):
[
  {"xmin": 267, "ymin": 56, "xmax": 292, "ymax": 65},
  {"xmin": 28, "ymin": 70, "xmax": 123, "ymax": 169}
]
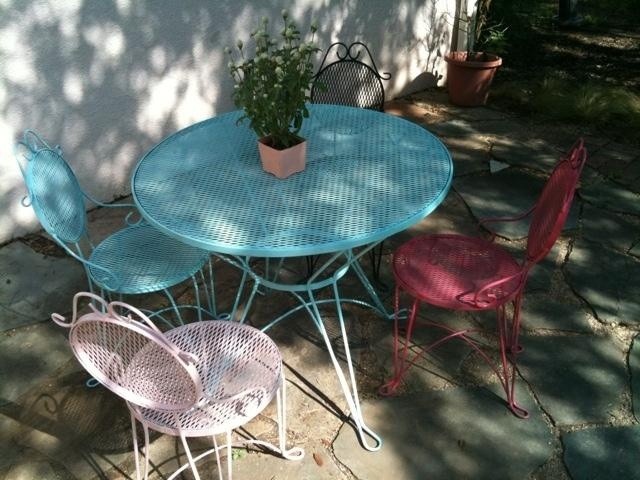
[{"xmin": 442, "ymin": 0, "xmax": 512, "ymax": 108}]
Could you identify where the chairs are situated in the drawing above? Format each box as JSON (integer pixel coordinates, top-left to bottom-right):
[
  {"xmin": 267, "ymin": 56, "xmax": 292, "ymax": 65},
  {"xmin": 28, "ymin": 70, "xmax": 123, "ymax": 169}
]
[
  {"xmin": 14, "ymin": 129, "xmax": 230, "ymax": 388},
  {"xmin": 51, "ymin": 292, "xmax": 303, "ymax": 474},
  {"xmin": 305, "ymin": 40, "xmax": 390, "ymax": 299},
  {"xmin": 379, "ymin": 138, "xmax": 590, "ymax": 422}
]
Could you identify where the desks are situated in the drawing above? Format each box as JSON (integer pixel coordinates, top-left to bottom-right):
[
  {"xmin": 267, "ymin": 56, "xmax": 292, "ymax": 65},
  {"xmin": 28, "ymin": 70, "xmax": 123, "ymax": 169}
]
[{"xmin": 131, "ymin": 105, "xmax": 455, "ymax": 453}]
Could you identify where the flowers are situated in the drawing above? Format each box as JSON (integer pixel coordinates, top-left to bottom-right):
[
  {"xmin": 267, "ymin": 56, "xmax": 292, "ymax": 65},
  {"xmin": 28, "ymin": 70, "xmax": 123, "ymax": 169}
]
[{"xmin": 224, "ymin": 9, "xmax": 326, "ymax": 146}]
